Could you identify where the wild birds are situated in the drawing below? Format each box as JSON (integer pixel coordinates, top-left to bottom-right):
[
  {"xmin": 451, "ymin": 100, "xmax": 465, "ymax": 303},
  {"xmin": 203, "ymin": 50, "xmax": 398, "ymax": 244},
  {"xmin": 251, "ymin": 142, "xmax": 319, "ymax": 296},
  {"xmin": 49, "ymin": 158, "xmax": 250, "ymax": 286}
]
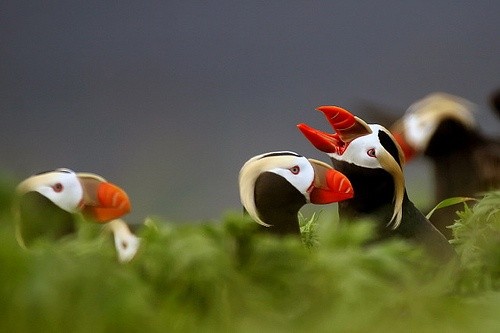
[
  {"xmin": 239, "ymin": 150, "xmax": 355, "ymax": 249},
  {"xmin": 13, "ymin": 168, "xmax": 133, "ymax": 259},
  {"xmin": 295, "ymin": 104, "xmax": 460, "ymax": 268}
]
